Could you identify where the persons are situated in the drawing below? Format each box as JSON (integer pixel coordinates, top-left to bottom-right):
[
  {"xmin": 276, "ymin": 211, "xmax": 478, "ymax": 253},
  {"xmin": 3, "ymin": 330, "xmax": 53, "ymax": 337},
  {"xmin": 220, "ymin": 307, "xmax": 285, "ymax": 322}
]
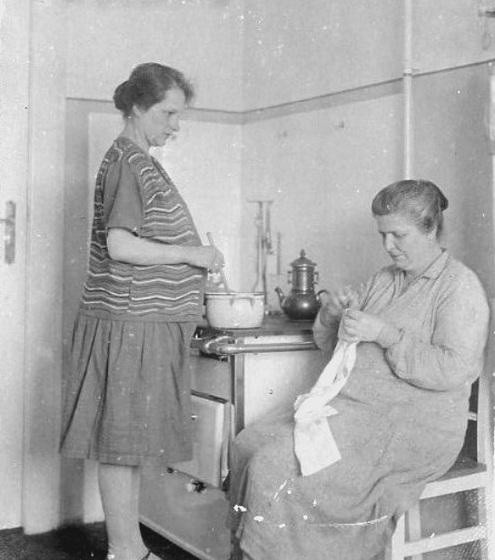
[
  {"xmin": 56, "ymin": 61, "xmax": 225, "ymax": 560},
  {"xmin": 224, "ymin": 179, "xmax": 491, "ymax": 559}
]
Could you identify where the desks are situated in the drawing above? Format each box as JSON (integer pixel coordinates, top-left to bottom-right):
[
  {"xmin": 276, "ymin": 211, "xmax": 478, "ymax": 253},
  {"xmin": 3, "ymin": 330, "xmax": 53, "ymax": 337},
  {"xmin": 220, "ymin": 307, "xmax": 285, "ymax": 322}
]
[{"xmin": 139, "ymin": 317, "xmax": 333, "ymax": 560}]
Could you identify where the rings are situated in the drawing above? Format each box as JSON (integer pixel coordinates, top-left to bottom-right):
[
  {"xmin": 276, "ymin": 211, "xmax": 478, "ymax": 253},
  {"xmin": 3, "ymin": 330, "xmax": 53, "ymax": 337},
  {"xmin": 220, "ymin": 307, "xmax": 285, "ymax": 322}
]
[{"xmin": 214, "ymin": 262, "xmax": 218, "ymax": 267}]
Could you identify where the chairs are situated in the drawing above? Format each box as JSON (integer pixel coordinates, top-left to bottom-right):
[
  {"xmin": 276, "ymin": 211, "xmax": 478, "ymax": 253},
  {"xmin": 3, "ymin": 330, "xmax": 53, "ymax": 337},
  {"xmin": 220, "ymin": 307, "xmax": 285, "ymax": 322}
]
[{"xmin": 376, "ymin": 296, "xmax": 495, "ymax": 560}]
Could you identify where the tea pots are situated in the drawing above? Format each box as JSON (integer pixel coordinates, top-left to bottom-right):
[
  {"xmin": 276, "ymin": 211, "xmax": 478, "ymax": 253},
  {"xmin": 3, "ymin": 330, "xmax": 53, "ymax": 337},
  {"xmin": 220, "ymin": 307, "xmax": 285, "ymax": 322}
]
[{"xmin": 274, "ymin": 249, "xmax": 331, "ymax": 322}]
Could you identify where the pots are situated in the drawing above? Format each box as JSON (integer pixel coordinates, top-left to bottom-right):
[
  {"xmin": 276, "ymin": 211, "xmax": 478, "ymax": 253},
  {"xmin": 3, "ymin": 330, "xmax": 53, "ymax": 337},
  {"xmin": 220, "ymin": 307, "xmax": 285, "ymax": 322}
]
[{"xmin": 206, "ymin": 293, "xmax": 264, "ymax": 329}]
